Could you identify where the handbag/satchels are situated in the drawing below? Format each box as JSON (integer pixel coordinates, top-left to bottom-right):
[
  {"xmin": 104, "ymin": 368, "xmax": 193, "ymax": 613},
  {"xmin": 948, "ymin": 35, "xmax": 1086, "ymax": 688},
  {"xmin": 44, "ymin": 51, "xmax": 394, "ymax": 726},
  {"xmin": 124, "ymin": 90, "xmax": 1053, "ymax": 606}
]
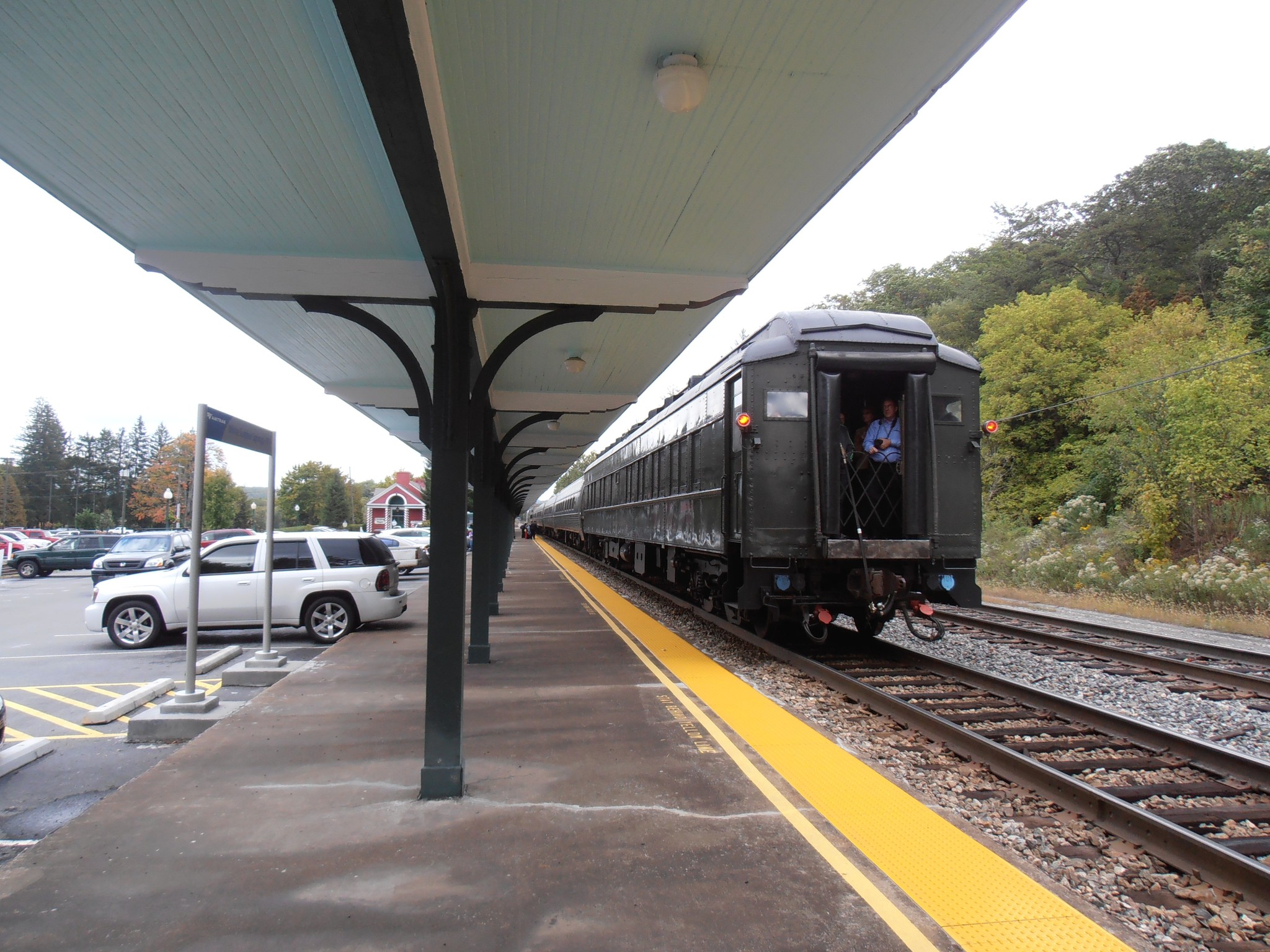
[{"xmin": 524, "ymin": 529, "xmax": 527, "ymax": 532}]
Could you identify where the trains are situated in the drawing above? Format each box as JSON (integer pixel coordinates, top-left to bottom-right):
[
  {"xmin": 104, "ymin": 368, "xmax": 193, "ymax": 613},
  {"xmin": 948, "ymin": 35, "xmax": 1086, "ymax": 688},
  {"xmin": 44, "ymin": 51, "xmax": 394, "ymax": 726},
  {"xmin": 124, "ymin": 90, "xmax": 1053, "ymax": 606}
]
[{"xmin": 529, "ymin": 308, "xmax": 999, "ymax": 646}]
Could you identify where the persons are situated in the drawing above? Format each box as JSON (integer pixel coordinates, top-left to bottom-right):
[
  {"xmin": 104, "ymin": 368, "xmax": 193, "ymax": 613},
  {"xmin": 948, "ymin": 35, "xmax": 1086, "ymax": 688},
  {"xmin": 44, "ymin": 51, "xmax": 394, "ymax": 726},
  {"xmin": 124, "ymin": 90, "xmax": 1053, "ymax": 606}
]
[
  {"xmin": 840, "ymin": 396, "xmax": 903, "ymax": 541},
  {"xmin": 154, "ymin": 536, "xmax": 168, "ymax": 550},
  {"xmin": 466, "ymin": 524, "xmax": 473, "ymax": 552},
  {"xmin": 932, "ymin": 396, "xmax": 959, "ymax": 422},
  {"xmin": 520, "ymin": 520, "xmax": 538, "ymax": 540}
]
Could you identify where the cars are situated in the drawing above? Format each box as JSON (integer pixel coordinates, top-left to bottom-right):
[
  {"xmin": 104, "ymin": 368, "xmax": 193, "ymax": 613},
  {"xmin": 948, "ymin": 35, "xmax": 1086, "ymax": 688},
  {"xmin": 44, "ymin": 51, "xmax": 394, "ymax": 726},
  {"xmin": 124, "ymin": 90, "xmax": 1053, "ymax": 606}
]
[
  {"xmin": 262, "ymin": 526, "xmax": 430, "ymax": 576},
  {"xmin": 0, "ymin": 526, "xmax": 98, "ymax": 558}
]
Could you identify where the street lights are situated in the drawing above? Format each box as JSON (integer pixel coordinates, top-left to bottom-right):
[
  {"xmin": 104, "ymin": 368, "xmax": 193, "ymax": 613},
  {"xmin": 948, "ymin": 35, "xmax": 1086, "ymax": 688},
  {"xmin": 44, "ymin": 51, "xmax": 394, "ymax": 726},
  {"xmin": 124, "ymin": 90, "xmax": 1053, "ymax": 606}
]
[
  {"xmin": 250, "ymin": 502, "xmax": 257, "ymax": 532},
  {"xmin": 294, "ymin": 504, "xmax": 301, "ymax": 527},
  {"xmin": 163, "ymin": 488, "xmax": 173, "ymax": 530}
]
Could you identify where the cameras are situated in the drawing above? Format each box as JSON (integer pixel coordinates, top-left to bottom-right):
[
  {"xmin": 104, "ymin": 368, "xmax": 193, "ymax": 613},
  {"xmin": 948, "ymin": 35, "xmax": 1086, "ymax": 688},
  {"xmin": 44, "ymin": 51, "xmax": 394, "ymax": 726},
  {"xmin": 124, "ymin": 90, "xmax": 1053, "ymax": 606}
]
[{"xmin": 874, "ymin": 439, "xmax": 885, "ymax": 451}]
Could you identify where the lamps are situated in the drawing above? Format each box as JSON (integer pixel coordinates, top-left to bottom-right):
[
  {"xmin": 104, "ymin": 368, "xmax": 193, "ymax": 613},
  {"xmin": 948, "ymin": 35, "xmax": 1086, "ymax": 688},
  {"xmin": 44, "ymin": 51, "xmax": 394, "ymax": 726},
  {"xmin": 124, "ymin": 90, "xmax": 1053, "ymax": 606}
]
[
  {"xmin": 547, "ymin": 421, "xmax": 560, "ymax": 431},
  {"xmin": 653, "ymin": 53, "xmax": 708, "ymax": 115},
  {"xmin": 564, "ymin": 357, "xmax": 587, "ymax": 375}
]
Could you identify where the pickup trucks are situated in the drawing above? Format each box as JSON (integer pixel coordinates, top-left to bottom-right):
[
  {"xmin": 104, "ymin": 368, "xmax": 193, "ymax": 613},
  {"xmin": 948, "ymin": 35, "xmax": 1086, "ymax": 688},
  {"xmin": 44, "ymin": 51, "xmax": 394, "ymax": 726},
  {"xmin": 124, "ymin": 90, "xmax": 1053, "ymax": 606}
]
[{"xmin": 107, "ymin": 527, "xmax": 134, "ymax": 535}]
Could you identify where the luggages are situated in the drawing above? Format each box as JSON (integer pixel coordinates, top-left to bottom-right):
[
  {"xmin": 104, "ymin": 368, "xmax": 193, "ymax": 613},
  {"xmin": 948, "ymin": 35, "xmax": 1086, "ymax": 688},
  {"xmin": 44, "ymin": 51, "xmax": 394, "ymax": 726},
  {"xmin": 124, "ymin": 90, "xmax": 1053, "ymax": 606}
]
[{"xmin": 525, "ymin": 532, "xmax": 528, "ymax": 538}]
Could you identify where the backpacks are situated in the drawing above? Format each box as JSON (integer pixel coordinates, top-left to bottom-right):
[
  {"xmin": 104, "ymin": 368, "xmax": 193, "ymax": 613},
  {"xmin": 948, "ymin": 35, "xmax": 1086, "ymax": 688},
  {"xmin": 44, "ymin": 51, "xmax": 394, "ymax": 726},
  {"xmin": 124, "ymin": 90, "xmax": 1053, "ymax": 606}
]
[{"xmin": 520, "ymin": 525, "xmax": 523, "ymax": 529}]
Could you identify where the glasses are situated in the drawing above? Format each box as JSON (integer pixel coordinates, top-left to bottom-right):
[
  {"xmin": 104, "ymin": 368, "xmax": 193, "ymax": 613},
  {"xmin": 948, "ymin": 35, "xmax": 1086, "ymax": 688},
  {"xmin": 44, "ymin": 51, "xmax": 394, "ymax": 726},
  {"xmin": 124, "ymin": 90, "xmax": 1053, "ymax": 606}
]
[{"xmin": 883, "ymin": 404, "xmax": 891, "ymax": 409}]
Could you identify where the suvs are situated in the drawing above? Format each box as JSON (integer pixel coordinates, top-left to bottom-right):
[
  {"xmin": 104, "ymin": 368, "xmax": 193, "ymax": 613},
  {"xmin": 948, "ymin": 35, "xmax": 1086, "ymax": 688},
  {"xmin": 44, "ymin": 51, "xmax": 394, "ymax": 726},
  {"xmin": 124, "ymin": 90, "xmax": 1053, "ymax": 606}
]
[
  {"xmin": 91, "ymin": 527, "xmax": 191, "ymax": 588},
  {"xmin": 83, "ymin": 531, "xmax": 408, "ymax": 650},
  {"xmin": 200, "ymin": 528, "xmax": 257, "ymax": 548},
  {"xmin": 6, "ymin": 533, "xmax": 125, "ymax": 578}
]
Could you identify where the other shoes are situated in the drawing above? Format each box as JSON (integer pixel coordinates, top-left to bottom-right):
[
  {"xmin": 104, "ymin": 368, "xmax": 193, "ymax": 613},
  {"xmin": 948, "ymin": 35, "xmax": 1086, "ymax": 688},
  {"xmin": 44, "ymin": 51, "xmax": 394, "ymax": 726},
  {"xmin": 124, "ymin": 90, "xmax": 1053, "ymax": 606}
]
[
  {"xmin": 533, "ymin": 538, "xmax": 536, "ymax": 540},
  {"xmin": 531, "ymin": 538, "xmax": 532, "ymax": 539}
]
